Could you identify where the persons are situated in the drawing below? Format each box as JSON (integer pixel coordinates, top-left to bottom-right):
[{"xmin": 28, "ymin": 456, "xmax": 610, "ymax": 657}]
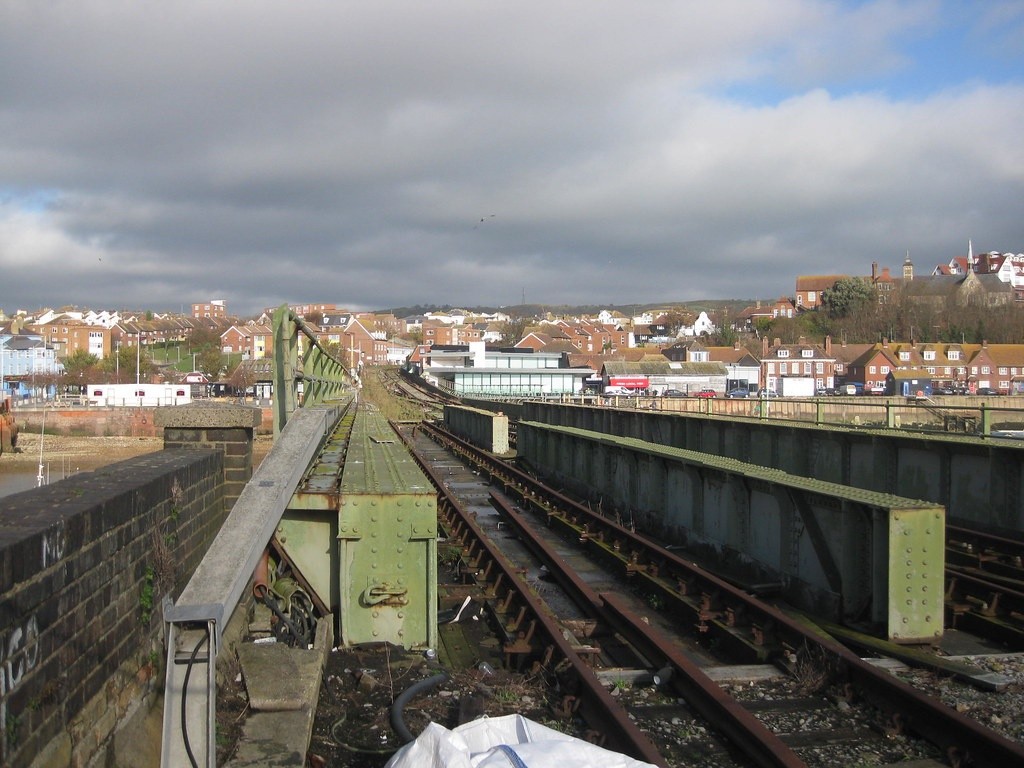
[{"xmin": 644, "ymin": 387, "xmax": 670, "ymax": 410}]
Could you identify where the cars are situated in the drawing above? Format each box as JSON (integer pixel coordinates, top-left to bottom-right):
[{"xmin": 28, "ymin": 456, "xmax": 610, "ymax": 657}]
[{"xmin": 583, "ymin": 382, "xmax": 885, "ymax": 397}]
